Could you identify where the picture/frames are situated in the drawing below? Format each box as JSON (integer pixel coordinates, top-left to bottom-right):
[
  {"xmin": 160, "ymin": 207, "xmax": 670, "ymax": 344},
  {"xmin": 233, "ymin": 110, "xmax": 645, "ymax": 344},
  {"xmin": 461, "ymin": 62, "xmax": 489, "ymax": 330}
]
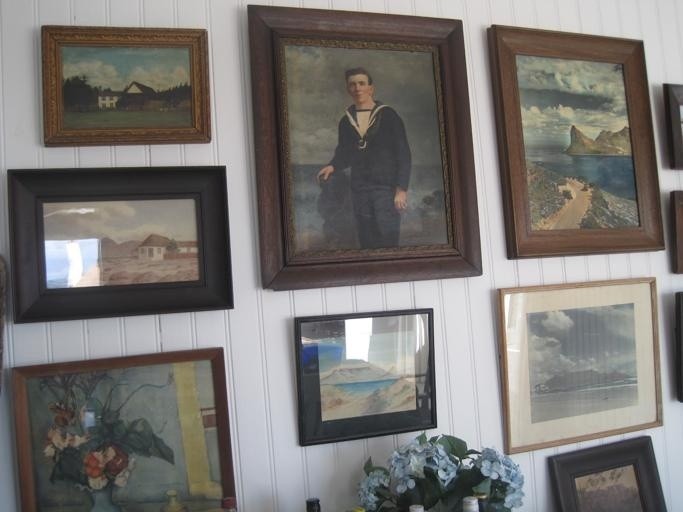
[
  {"xmin": 246, "ymin": 3, "xmax": 484, "ymax": 291},
  {"xmin": 6, "ymin": 164, "xmax": 236, "ymax": 324},
  {"xmin": 669, "ymin": 190, "xmax": 682, "ymax": 274},
  {"xmin": 490, "ymin": 23, "xmax": 665, "ymax": 260},
  {"xmin": 674, "ymin": 291, "xmax": 683, "ymax": 402},
  {"xmin": 498, "ymin": 276, "xmax": 664, "ymax": 455},
  {"xmin": 547, "ymin": 435, "xmax": 668, "ymax": 512},
  {"xmin": 663, "ymin": 81, "xmax": 683, "ymax": 172},
  {"xmin": 10, "ymin": 346, "xmax": 237, "ymax": 512},
  {"xmin": 39, "ymin": 23, "xmax": 215, "ymax": 149},
  {"xmin": 294, "ymin": 308, "xmax": 438, "ymax": 446}
]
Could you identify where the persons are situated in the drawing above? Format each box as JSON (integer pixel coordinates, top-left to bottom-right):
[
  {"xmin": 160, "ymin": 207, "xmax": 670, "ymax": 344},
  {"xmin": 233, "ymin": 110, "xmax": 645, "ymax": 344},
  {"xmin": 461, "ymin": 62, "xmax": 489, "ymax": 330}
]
[{"xmin": 316, "ymin": 65, "xmax": 412, "ymax": 250}]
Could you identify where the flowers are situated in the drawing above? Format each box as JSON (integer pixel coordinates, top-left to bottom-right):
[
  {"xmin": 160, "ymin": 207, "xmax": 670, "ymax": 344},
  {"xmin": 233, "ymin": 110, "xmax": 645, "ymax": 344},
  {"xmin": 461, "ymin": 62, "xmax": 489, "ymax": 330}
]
[
  {"xmin": 356, "ymin": 432, "xmax": 525, "ymax": 512},
  {"xmin": 39, "ymin": 369, "xmax": 175, "ymax": 488}
]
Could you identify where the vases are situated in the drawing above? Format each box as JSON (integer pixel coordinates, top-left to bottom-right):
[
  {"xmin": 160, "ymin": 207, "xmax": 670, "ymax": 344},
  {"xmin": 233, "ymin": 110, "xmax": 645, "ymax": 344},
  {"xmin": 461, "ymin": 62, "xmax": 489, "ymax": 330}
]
[
  {"xmin": 160, "ymin": 488, "xmax": 188, "ymax": 512},
  {"xmin": 75, "ymin": 481, "xmax": 125, "ymax": 511}
]
